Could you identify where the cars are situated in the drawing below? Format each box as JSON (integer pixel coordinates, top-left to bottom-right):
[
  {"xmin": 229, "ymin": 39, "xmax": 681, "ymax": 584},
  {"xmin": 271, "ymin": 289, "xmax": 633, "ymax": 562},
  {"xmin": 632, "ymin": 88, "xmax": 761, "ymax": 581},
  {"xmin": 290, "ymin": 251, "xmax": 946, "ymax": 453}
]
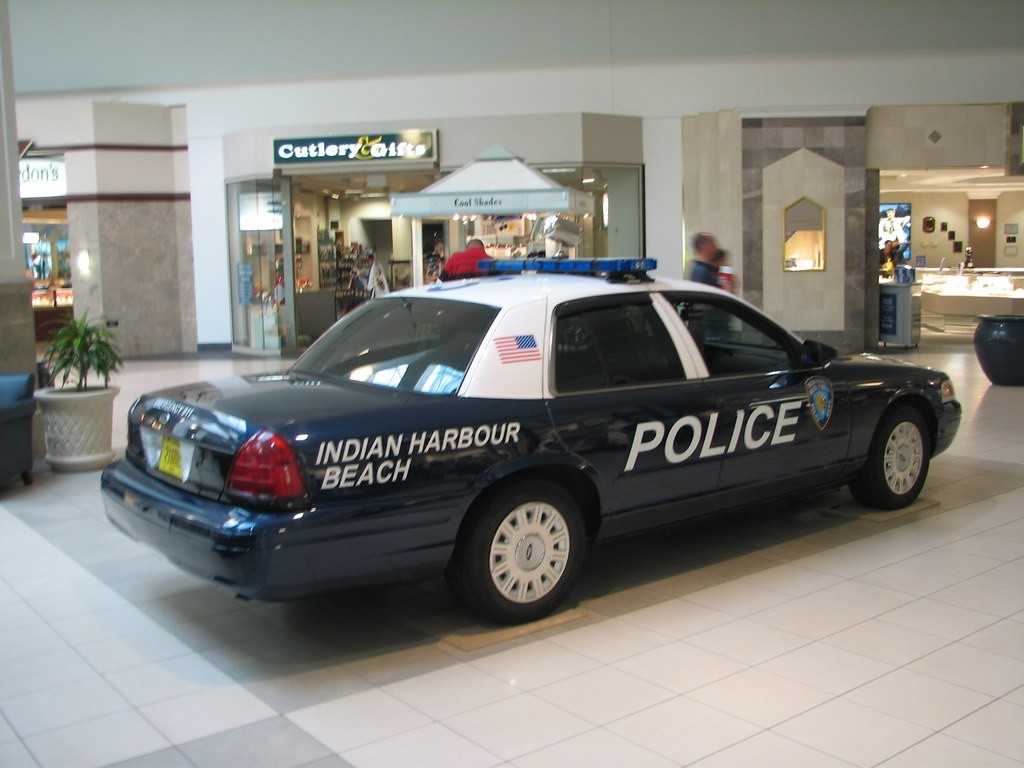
[{"xmin": 101, "ymin": 257, "xmax": 962, "ymax": 621}]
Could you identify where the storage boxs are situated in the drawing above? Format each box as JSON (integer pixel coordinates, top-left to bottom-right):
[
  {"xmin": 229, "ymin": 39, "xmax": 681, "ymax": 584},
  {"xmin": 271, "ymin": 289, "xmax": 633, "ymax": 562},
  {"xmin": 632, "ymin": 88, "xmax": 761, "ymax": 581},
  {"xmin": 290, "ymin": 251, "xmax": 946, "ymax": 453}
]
[{"xmin": 973, "ymin": 313, "xmax": 1024, "ymax": 387}]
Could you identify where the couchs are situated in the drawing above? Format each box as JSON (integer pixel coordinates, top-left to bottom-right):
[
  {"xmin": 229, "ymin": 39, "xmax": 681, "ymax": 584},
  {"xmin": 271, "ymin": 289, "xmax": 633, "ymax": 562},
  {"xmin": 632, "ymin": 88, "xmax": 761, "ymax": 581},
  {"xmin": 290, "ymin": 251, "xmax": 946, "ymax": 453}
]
[{"xmin": 0, "ymin": 373, "xmax": 39, "ymax": 497}]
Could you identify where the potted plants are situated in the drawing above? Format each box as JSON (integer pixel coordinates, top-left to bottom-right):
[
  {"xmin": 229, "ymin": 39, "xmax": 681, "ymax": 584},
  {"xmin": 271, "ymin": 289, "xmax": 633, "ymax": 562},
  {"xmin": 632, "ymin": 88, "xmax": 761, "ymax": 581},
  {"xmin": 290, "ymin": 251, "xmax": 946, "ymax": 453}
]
[{"xmin": 33, "ymin": 306, "xmax": 124, "ymax": 474}]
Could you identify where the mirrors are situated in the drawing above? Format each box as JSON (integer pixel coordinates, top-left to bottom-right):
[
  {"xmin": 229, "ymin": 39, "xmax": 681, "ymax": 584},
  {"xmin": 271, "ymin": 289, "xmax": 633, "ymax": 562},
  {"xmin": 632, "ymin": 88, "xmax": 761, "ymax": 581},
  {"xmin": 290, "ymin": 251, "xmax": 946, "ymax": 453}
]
[{"xmin": 782, "ymin": 195, "xmax": 827, "ymax": 272}]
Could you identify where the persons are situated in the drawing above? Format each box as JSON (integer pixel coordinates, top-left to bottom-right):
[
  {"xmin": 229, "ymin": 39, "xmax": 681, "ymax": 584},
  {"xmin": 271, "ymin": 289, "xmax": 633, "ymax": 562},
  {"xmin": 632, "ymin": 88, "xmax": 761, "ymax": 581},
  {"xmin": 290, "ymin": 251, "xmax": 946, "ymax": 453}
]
[
  {"xmin": 684, "ymin": 231, "xmax": 721, "ymax": 342},
  {"xmin": 436, "ymin": 239, "xmax": 495, "ymax": 284},
  {"xmin": 879, "ymin": 208, "xmax": 911, "ymax": 249},
  {"xmin": 714, "ymin": 249, "xmax": 726, "ymax": 288},
  {"xmin": 890, "ymin": 241, "xmax": 910, "ymax": 266},
  {"xmin": 426, "ymin": 241, "xmax": 442, "ymax": 286},
  {"xmin": 878, "ymin": 240, "xmax": 892, "ymax": 267}
]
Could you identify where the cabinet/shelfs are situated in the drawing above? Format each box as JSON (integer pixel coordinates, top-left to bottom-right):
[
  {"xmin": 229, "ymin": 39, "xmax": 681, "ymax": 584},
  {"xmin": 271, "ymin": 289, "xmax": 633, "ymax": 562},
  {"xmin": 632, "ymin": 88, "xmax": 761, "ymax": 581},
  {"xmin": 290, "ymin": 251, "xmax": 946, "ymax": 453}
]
[{"xmin": 880, "ymin": 282, "xmax": 922, "ymax": 350}]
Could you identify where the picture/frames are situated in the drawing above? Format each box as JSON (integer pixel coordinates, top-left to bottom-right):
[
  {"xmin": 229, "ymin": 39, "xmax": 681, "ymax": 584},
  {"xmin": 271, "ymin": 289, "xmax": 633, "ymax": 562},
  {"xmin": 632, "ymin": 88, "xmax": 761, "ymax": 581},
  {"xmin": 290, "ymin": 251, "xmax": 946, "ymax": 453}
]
[{"xmin": 877, "ymin": 203, "xmax": 912, "ymax": 261}]
